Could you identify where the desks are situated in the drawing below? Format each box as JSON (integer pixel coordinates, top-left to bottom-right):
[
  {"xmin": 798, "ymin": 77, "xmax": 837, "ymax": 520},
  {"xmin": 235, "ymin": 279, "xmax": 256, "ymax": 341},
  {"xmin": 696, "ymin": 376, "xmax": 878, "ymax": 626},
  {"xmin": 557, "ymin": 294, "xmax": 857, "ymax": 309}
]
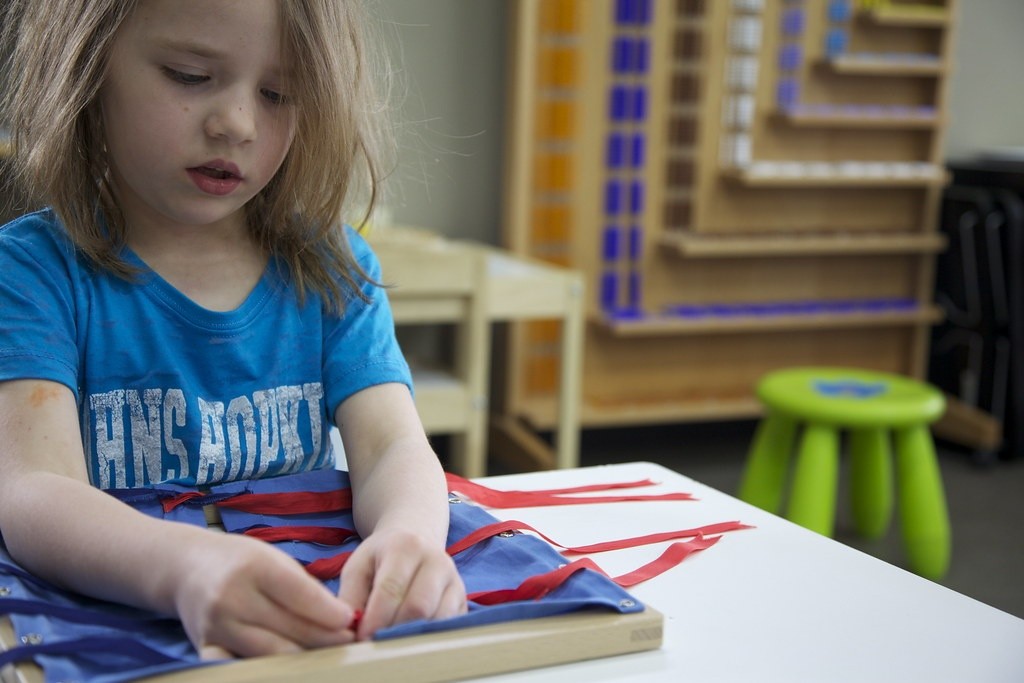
[{"xmin": 365, "ymin": 221, "xmax": 1024, "ymax": 683}]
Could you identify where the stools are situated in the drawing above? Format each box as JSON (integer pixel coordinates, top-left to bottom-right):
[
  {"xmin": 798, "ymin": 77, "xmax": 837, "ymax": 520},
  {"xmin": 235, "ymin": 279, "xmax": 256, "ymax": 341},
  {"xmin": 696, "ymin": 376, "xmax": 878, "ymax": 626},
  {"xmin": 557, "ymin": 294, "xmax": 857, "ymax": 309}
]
[{"xmin": 738, "ymin": 369, "xmax": 952, "ymax": 583}]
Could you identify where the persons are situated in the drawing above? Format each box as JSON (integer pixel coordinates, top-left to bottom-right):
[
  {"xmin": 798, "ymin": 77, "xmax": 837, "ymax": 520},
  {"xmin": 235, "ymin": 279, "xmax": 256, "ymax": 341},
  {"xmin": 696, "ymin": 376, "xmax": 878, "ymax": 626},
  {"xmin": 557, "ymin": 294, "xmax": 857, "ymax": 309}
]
[{"xmin": 0, "ymin": 0, "xmax": 469, "ymax": 662}]
